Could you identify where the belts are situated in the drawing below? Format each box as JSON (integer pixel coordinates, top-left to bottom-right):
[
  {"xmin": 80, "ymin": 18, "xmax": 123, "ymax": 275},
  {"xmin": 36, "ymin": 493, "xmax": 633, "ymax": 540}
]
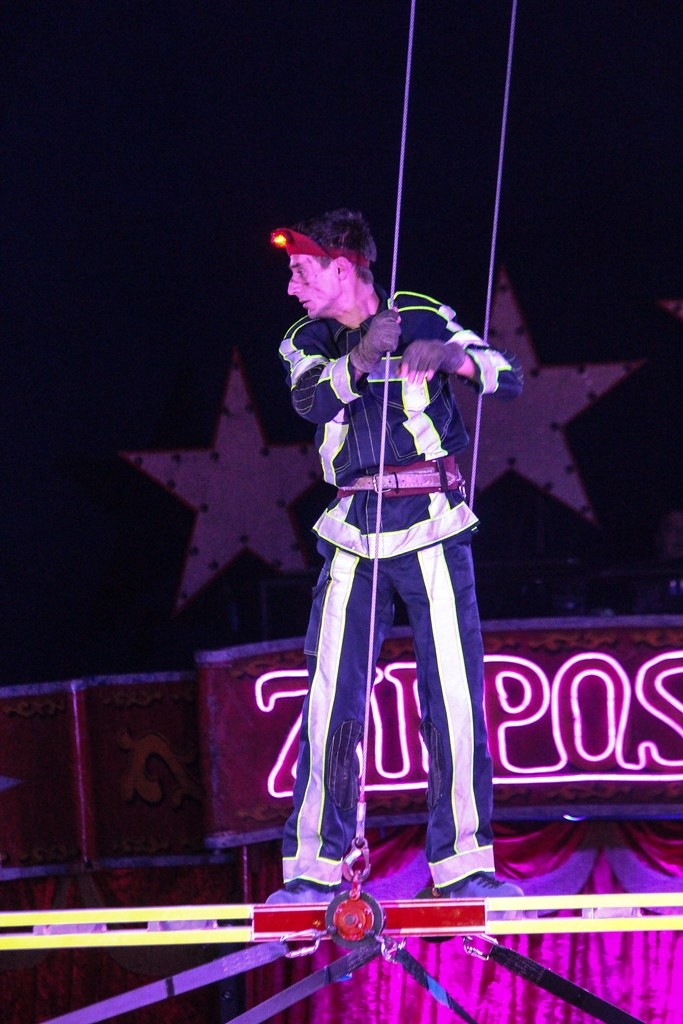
[{"xmin": 338, "ymin": 466, "xmax": 457, "ymax": 494}]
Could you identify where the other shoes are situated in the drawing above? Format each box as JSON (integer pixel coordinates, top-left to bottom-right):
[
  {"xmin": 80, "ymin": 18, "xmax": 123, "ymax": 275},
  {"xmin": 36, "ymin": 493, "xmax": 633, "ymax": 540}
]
[
  {"xmin": 450, "ymin": 871, "xmax": 524, "ymax": 896},
  {"xmin": 264, "ymin": 883, "xmax": 334, "ymax": 904}
]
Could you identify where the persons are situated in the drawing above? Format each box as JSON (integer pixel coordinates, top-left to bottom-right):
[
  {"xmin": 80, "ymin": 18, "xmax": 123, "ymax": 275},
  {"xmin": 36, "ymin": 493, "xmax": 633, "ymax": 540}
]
[{"xmin": 266, "ymin": 208, "xmax": 526, "ymax": 903}]
